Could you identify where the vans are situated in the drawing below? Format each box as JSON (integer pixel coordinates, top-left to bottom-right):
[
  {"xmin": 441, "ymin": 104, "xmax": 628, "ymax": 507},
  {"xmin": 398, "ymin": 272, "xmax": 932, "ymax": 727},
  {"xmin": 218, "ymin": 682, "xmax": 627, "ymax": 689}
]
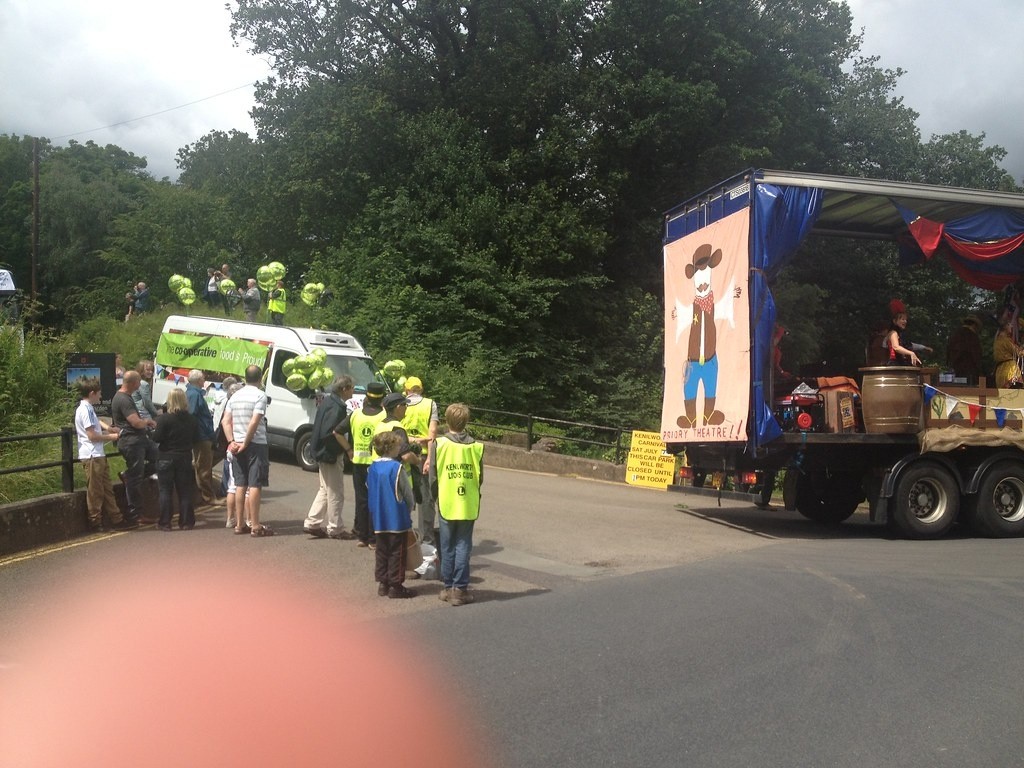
[{"xmin": 151, "ymin": 315, "xmax": 393, "ymax": 472}]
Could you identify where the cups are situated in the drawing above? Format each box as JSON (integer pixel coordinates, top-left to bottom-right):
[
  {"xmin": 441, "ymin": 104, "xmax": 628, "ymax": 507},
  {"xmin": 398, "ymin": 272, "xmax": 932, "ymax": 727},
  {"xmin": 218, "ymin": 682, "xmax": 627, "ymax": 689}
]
[{"xmin": 945, "ymin": 374, "xmax": 954, "ymax": 381}]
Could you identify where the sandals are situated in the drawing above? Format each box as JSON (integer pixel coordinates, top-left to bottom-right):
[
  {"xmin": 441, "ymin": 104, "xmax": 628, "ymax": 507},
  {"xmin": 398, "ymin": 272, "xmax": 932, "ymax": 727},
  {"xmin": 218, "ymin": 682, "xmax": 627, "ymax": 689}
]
[
  {"xmin": 234, "ymin": 524, "xmax": 252, "ymax": 534},
  {"xmin": 251, "ymin": 526, "xmax": 273, "ymax": 536}
]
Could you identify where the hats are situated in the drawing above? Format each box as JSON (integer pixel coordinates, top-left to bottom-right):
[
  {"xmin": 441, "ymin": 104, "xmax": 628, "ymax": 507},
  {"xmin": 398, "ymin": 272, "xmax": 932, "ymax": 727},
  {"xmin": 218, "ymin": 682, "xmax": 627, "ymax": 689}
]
[
  {"xmin": 960, "ymin": 313, "xmax": 982, "ymax": 331},
  {"xmin": 383, "ymin": 393, "xmax": 411, "ymax": 409},
  {"xmin": 366, "ymin": 383, "xmax": 386, "ymax": 400},
  {"xmin": 405, "ymin": 376, "xmax": 422, "ymax": 390}
]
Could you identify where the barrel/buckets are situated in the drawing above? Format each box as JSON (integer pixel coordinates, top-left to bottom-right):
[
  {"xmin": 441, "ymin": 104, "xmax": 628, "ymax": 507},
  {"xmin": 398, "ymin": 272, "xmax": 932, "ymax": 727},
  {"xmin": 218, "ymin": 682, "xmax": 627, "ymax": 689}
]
[{"xmin": 407, "ymin": 527, "xmax": 424, "ymax": 570}]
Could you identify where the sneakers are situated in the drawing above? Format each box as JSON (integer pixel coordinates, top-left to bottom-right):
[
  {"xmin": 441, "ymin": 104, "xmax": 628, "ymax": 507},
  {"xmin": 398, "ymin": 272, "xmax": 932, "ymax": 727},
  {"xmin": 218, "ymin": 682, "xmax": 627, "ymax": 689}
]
[
  {"xmin": 451, "ymin": 587, "xmax": 474, "ymax": 605},
  {"xmin": 378, "ymin": 584, "xmax": 388, "ymax": 595},
  {"xmin": 388, "ymin": 585, "xmax": 416, "ymax": 598},
  {"xmin": 438, "ymin": 588, "xmax": 452, "ymax": 601}
]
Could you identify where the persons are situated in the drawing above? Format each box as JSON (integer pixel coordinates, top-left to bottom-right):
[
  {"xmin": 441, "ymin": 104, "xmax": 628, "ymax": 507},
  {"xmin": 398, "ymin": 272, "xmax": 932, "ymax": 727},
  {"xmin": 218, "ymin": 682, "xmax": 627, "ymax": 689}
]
[
  {"xmin": 369, "ymin": 393, "xmax": 422, "ymax": 579},
  {"xmin": 206, "ymin": 263, "xmax": 233, "ymax": 316},
  {"xmin": 993, "ymin": 317, "xmax": 1024, "ymax": 389},
  {"xmin": 363, "ymin": 431, "xmax": 417, "ymax": 598},
  {"xmin": 238, "ymin": 279, "xmax": 261, "ymax": 322},
  {"xmin": 753, "ymin": 470, "xmax": 777, "ymax": 511},
  {"xmin": 947, "ymin": 314, "xmax": 982, "ymax": 385},
  {"xmin": 868, "ymin": 299, "xmax": 933, "ymax": 366},
  {"xmin": 429, "ymin": 403, "xmax": 484, "ymax": 605},
  {"xmin": 302, "ymin": 376, "xmax": 440, "ymax": 547},
  {"xmin": 267, "ymin": 281, "xmax": 286, "ymax": 325},
  {"xmin": 75, "ymin": 355, "xmax": 276, "ymax": 537},
  {"xmin": 126, "ymin": 282, "xmax": 149, "ymax": 315}
]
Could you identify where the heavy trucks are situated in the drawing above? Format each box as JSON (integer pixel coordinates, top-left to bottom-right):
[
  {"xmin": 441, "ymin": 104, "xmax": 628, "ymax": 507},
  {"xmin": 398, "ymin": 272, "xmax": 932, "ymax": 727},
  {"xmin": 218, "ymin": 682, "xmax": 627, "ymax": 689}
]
[{"xmin": 661, "ymin": 169, "xmax": 1023, "ymax": 537}]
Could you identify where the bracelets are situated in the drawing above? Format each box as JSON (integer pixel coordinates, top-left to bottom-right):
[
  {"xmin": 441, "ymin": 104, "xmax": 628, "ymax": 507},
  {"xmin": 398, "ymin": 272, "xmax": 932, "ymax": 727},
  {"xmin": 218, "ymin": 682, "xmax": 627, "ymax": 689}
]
[{"xmin": 228, "ymin": 440, "xmax": 234, "ymax": 444}]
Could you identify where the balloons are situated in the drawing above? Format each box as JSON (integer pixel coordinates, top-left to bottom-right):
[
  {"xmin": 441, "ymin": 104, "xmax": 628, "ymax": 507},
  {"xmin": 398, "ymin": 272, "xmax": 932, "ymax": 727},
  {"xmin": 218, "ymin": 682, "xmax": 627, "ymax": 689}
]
[
  {"xmin": 301, "ymin": 282, "xmax": 325, "ymax": 307},
  {"xmin": 256, "ymin": 261, "xmax": 286, "ymax": 291},
  {"xmin": 168, "ymin": 275, "xmax": 196, "ymax": 305},
  {"xmin": 375, "ymin": 359, "xmax": 408, "ymax": 391},
  {"xmin": 282, "ymin": 347, "xmax": 334, "ymax": 391},
  {"xmin": 218, "ymin": 279, "xmax": 235, "ymax": 296}
]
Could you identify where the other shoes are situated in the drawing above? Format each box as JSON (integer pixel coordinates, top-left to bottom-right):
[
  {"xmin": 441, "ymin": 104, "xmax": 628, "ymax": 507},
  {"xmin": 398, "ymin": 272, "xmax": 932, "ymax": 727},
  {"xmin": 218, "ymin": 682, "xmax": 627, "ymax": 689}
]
[
  {"xmin": 329, "ymin": 530, "xmax": 354, "ymax": 540},
  {"xmin": 303, "ymin": 526, "xmax": 328, "ymax": 537},
  {"xmin": 368, "ymin": 542, "xmax": 377, "ymax": 550},
  {"xmin": 357, "ymin": 540, "xmax": 368, "ymax": 547},
  {"xmin": 128, "ymin": 515, "xmax": 158, "ymax": 524},
  {"xmin": 155, "ymin": 521, "xmax": 173, "ymax": 531},
  {"xmin": 90, "ymin": 524, "xmax": 107, "ymax": 532},
  {"xmin": 112, "ymin": 519, "xmax": 138, "ymax": 531},
  {"xmin": 246, "ymin": 521, "xmax": 268, "ymax": 529},
  {"xmin": 118, "ymin": 471, "xmax": 126, "ymax": 485},
  {"xmin": 226, "ymin": 519, "xmax": 236, "ymax": 527},
  {"xmin": 179, "ymin": 524, "xmax": 193, "ymax": 531},
  {"xmin": 404, "ymin": 571, "xmax": 420, "ymax": 579}
]
